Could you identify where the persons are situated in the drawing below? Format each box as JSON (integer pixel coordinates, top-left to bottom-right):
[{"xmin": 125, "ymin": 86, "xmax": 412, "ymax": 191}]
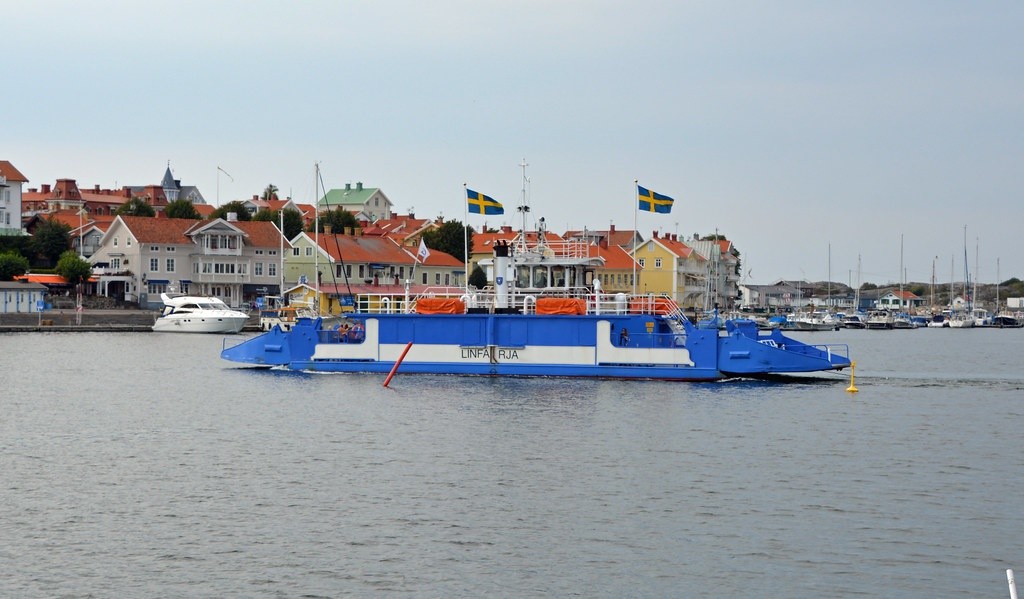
[
  {"xmin": 618, "ymin": 328, "xmax": 631, "ymax": 346},
  {"xmin": 535, "ymin": 272, "xmax": 547, "ymax": 287},
  {"xmin": 328, "ymin": 321, "xmax": 365, "ymax": 342}
]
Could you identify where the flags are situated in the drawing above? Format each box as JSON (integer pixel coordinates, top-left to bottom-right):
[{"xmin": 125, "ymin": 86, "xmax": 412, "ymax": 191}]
[
  {"xmin": 467, "ymin": 188, "xmax": 504, "ymax": 215},
  {"xmin": 419, "ymin": 239, "xmax": 430, "ymax": 262},
  {"xmin": 638, "ymin": 185, "xmax": 674, "ymax": 214}
]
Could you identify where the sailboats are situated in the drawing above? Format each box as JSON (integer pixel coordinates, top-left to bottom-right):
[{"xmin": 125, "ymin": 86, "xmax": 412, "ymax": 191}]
[{"xmin": 697, "ymin": 226, "xmax": 1024, "ymax": 332}]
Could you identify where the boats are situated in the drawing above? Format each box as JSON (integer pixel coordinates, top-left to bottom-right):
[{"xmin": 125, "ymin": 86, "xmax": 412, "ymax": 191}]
[
  {"xmin": 151, "ymin": 292, "xmax": 250, "ymax": 334},
  {"xmin": 221, "ymin": 158, "xmax": 861, "ymax": 393},
  {"xmin": 259, "ymin": 307, "xmax": 348, "ymax": 332}
]
